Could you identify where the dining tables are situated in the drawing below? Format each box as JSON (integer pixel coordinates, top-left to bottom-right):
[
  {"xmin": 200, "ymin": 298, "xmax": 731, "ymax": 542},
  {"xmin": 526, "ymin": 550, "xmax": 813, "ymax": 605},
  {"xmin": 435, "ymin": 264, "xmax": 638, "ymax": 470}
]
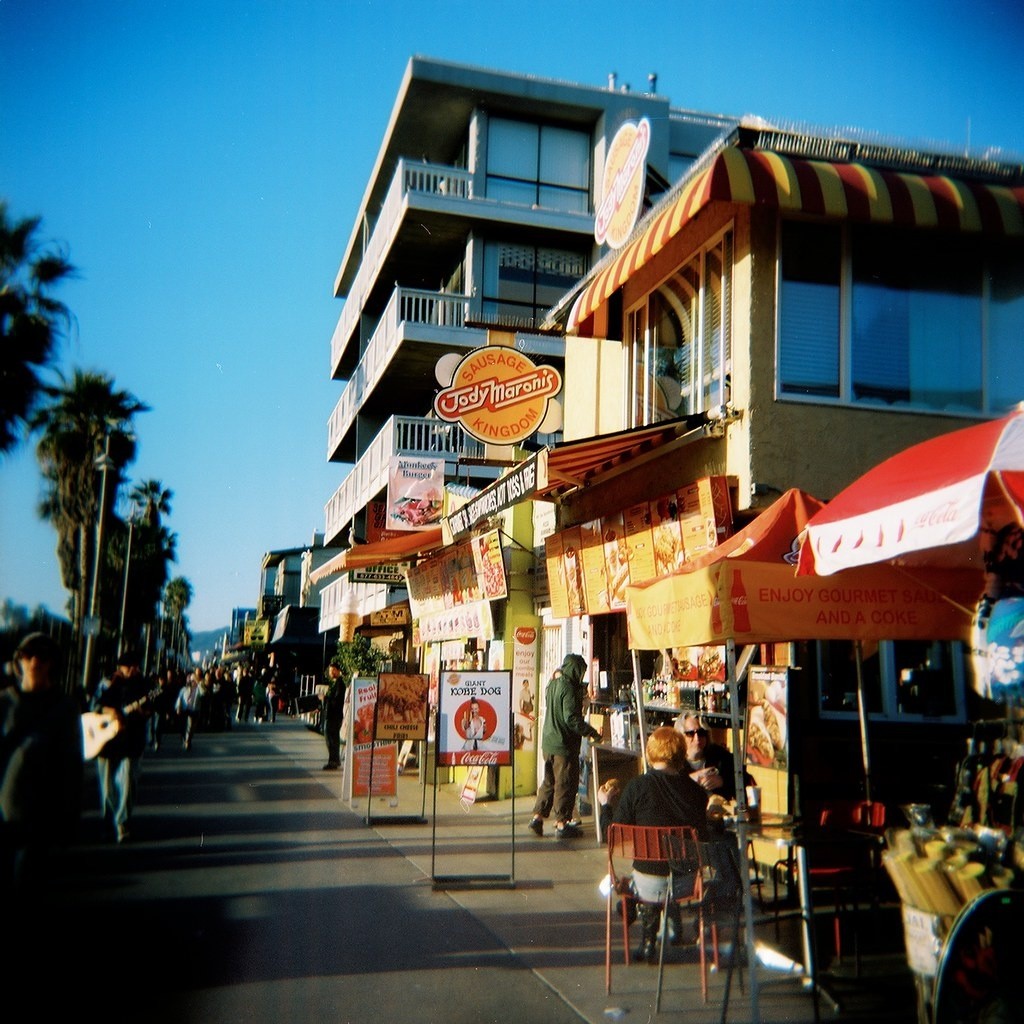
[{"xmin": 716, "ymin": 808, "xmax": 796, "ymax": 904}]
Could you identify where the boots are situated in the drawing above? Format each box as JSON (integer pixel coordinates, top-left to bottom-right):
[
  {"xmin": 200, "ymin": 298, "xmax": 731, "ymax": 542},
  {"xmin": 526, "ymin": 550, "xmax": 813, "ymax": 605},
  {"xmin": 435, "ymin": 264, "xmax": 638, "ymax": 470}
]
[{"xmin": 633, "ymin": 904, "xmax": 661, "ymax": 960}]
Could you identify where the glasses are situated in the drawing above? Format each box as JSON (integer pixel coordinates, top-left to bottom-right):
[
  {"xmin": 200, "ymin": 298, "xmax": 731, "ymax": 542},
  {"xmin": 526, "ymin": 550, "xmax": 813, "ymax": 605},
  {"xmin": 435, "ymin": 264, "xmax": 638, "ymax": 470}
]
[
  {"xmin": 684, "ymin": 727, "xmax": 709, "ymax": 737},
  {"xmin": 16, "ymin": 646, "xmax": 55, "ymax": 661}
]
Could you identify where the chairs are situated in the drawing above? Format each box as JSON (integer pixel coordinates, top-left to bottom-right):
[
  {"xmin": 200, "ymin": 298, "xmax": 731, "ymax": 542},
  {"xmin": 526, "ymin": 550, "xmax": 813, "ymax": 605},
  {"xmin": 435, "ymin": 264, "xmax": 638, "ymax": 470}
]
[
  {"xmin": 601, "ymin": 822, "xmax": 726, "ymax": 1005},
  {"xmin": 805, "ymin": 795, "xmax": 892, "ymax": 956}
]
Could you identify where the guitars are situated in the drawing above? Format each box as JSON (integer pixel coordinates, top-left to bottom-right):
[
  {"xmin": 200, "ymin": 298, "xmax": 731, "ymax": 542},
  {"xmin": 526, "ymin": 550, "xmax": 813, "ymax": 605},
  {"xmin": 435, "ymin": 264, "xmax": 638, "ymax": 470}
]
[{"xmin": 80, "ymin": 686, "xmax": 165, "ymax": 762}]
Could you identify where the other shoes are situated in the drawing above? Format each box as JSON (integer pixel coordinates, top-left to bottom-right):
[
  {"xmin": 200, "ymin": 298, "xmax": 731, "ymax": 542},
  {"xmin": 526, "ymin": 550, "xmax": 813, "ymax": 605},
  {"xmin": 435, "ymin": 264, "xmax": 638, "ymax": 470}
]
[
  {"xmin": 555, "ymin": 823, "xmax": 584, "ymax": 839},
  {"xmin": 528, "ymin": 817, "xmax": 544, "ymax": 837},
  {"xmin": 322, "ymin": 761, "xmax": 341, "ymax": 771},
  {"xmin": 668, "ymin": 932, "xmax": 683, "ymax": 946},
  {"xmin": 116, "ymin": 825, "xmax": 129, "ymax": 843}
]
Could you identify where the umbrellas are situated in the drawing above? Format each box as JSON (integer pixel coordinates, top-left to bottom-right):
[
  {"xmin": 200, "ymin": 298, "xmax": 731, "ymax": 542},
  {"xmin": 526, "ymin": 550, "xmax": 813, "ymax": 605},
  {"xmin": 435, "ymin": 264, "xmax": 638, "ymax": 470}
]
[{"xmin": 797, "ymin": 397, "xmax": 1024, "ymax": 569}]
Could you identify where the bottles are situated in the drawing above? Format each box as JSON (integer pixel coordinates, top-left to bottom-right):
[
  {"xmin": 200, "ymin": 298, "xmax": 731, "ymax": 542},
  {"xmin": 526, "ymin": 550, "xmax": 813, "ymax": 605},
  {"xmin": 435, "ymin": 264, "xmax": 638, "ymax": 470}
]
[
  {"xmin": 642, "ymin": 674, "xmax": 727, "ymax": 712},
  {"xmin": 617, "ymin": 678, "xmax": 636, "ymax": 705}
]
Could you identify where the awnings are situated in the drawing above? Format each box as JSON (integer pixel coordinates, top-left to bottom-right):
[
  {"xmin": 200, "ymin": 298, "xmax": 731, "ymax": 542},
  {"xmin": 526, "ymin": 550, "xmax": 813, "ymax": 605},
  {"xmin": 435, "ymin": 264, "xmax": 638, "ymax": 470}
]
[
  {"xmin": 310, "ymin": 527, "xmax": 442, "ymax": 585},
  {"xmin": 449, "ymin": 404, "xmax": 726, "ymax": 537}
]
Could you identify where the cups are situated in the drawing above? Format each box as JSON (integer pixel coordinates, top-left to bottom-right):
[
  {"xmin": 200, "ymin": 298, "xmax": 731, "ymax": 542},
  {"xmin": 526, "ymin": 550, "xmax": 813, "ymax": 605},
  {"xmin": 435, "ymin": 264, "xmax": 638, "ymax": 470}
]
[
  {"xmin": 563, "ymin": 546, "xmax": 575, "ymax": 584},
  {"xmin": 604, "ymin": 530, "xmax": 620, "ymax": 577},
  {"xmin": 746, "ymin": 785, "xmax": 761, "ymax": 817}
]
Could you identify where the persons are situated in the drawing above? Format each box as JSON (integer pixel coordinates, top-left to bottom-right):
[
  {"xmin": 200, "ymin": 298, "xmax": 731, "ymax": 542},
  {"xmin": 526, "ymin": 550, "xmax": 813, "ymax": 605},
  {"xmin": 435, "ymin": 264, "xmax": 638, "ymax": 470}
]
[
  {"xmin": 321, "ymin": 663, "xmax": 345, "ymax": 771},
  {"xmin": 528, "ymin": 654, "xmax": 603, "ymax": 839},
  {"xmin": 598, "ymin": 725, "xmax": 708, "ymax": 958},
  {"xmin": 2, "ymin": 634, "xmax": 62, "ymax": 752},
  {"xmin": 157, "ymin": 665, "xmax": 281, "ymax": 751},
  {"xmin": 89, "ymin": 653, "xmax": 153, "ymax": 845},
  {"xmin": 673, "ymin": 713, "xmax": 757, "ymax": 838}
]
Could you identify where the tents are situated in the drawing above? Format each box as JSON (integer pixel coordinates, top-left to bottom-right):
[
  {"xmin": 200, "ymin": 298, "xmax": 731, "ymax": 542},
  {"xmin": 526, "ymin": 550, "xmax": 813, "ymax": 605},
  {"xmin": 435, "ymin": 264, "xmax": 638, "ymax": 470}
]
[{"xmin": 622, "ymin": 488, "xmax": 986, "ymax": 1021}]
